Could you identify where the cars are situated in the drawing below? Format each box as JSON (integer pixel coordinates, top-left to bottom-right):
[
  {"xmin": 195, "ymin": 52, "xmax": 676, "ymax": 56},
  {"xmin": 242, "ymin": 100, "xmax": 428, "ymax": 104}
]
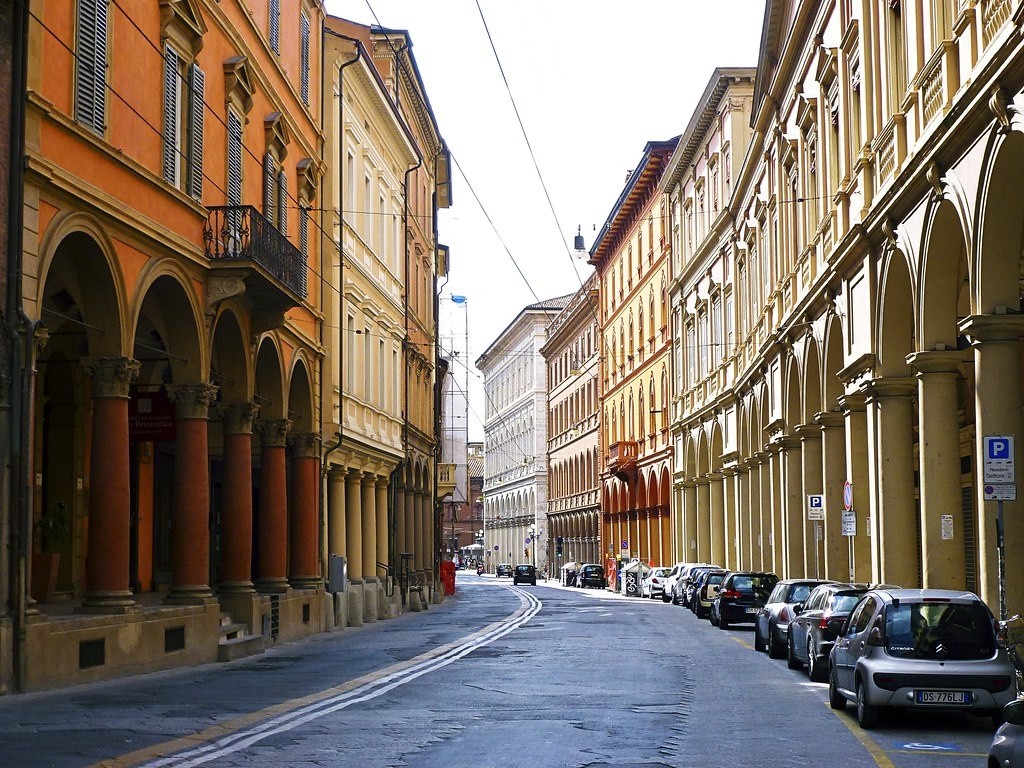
[
  {"xmin": 511, "ymin": 564, "xmax": 537, "ymax": 586},
  {"xmin": 755, "ymin": 579, "xmax": 841, "ymax": 659},
  {"xmin": 709, "ymin": 571, "xmax": 780, "ymax": 629},
  {"xmin": 787, "ymin": 582, "xmax": 928, "ymax": 682},
  {"xmin": 641, "ymin": 567, "xmax": 673, "ymax": 599},
  {"xmin": 662, "ymin": 563, "xmax": 731, "ymax": 619},
  {"xmin": 829, "ymin": 589, "xmax": 1017, "ymax": 729},
  {"xmin": 988, "ymin": 700, "xmax": 1024, "ymax": 768},
  {"xmin": 576, "ymin": 564, "xmax": 605, "ymax": 589},
  {"xmin": 495, "ymin": 564, "xmax": 513, "ymax": 577}
]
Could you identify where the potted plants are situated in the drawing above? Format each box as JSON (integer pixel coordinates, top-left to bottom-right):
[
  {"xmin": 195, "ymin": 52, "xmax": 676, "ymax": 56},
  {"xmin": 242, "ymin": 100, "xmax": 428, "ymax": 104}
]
[{"xmin": 35, "ymin": 504, "xmax": 69, "ymax": 600}]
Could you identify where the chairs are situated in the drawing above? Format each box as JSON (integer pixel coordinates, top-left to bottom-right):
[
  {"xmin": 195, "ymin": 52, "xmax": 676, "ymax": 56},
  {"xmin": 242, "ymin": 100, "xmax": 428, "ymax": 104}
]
[
  {"xmin": 892, "ymin": 611, "xmax": 929, "ymax": 635},
  {"xmin": 938, "ymin": 606, "xmax": 971, "ymax": 629}
]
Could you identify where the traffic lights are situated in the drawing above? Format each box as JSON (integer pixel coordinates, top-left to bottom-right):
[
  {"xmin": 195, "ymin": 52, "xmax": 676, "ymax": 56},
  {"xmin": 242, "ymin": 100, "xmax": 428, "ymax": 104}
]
[
  {"xmin": 524, "ymin": 548, "xmax": 530, "ymax": 557},
  {"xmin": 556, "ymin": 547, "xmax": 562, "ymax": 557},
  {"xmin": 557, "ymin": 538, "xmax": 563, "ymax": 545}
]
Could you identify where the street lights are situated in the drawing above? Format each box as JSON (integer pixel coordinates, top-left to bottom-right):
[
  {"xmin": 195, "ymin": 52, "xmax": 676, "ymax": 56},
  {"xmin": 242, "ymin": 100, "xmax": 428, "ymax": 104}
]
[
  {"xmin": 475, "ymin": 529, "xmax": 483, "ymax": 560},
  {"xmin": 527, "ymin": 524, "xmax": 542, "ymax": 567}
]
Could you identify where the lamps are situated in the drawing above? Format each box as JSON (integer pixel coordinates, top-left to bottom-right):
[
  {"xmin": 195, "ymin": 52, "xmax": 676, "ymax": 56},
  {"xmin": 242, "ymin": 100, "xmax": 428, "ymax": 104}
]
[
  {"xmin": 521, "ymin": 459, "xmax": 530, "ymax": 467},
  {"xmin": 570, "ymin": 423, "xmax": 579, "ymax": 435},
  {"xmin": 571, "ymin": 358, "xmax": 583, "ymax": 377},
  {"xmin": 571, "ymin": 224, "xmax": 587, "ymax": 259}
]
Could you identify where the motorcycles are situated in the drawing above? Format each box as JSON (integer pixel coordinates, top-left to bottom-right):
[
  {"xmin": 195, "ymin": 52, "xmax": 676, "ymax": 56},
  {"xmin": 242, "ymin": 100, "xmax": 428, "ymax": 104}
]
[{"xmin": 477, "ymin": 567, "xmax": 482, "ymax": 576}]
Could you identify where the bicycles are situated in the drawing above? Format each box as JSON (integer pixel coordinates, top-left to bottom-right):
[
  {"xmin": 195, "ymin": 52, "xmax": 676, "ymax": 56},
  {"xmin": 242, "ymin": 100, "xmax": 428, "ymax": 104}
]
[{"xmin": 999, "ymin": 614, "xmax": 1024, "ymax": 677}]
